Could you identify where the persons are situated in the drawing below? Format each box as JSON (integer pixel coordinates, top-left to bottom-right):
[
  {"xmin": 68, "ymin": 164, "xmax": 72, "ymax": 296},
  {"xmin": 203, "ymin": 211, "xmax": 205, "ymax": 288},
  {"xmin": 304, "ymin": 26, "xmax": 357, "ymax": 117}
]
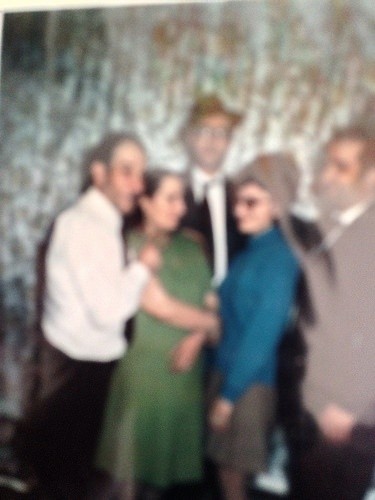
[
  {"xmin": 95, "ymin": 166, "xmax": 222, "ymax": 500},
  {"xmin": 172, "ymin": 92, "xmax": 253, "ymax": 276},
  {"xmin": 25, "ymin": 130, "xmax": 166, "ymax": 500},
  {"xmin": 279, "ymin": 128, "xmax": 375, "ymax": 500},
  {"xmin": 202, "ymin": 149, "xmax": 308, "ymax": 500}
]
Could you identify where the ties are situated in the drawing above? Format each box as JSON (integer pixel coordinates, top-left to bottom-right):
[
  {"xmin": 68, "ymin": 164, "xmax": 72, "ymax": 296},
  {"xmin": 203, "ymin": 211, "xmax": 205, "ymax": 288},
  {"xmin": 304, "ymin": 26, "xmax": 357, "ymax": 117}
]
[{"xmin": 199, "ymin": 185, "xmax": 216, "ymax": 277}]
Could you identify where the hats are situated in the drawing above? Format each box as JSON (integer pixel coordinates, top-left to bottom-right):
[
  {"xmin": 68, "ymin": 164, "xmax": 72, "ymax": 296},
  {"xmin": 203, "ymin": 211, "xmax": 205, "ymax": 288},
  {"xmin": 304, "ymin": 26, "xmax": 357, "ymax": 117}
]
[
  {"xmin": 178, "ymin": 94, "xmax": 242, "ymax": 126},
  {"xmin": 234, "ymin": 153, "xmax": 299, "ymax": 203}
]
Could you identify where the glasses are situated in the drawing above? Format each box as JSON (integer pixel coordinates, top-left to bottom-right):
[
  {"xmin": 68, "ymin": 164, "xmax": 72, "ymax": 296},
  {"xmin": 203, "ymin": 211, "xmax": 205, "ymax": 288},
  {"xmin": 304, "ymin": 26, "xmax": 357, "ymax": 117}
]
[{"xmin": 235, "ymin": 198, "xmax": 259, "ymax": 207}]
